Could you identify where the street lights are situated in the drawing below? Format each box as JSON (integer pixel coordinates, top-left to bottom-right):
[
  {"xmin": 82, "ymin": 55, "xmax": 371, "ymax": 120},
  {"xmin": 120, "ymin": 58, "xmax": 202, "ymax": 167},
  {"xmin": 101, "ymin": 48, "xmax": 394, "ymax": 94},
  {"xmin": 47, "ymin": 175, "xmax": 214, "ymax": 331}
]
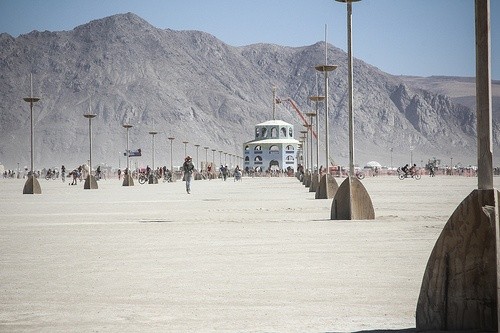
[
  {"xmin": 315, "ymin": 64, "xmax": 339, "ymax": 200},
  {"xmin": 295, "ymin": 94, "xmax": 326, "ymax": 195},
  {"xmin": 121, "ymin": 125, "xmax": 135, "ymax": 185},
  {"xmin": 22, "ymin": 99, "xmax": 43, "ymax": 195},
  {"xmin": 168, "ymin": 137, "xmax": 177, "ymax": 182},
  {"xmin": 146, "ymin": 131, "xmax": 158, "ymax": 184},
  {"xmin": 84, "ymin": 113, "xmax": 99, "ymax": 190},
  {"xmin": 182, "ymin": 141, "xmax": 243, "ymax": 180}
]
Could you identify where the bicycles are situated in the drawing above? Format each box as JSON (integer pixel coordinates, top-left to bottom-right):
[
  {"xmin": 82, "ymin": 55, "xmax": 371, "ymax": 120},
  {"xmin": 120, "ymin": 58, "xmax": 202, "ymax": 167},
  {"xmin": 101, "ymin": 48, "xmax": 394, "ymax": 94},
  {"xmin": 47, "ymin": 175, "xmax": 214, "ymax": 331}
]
[{"xmin": 398, "ymin": 169, "xmax": 422, "ymax": 180}]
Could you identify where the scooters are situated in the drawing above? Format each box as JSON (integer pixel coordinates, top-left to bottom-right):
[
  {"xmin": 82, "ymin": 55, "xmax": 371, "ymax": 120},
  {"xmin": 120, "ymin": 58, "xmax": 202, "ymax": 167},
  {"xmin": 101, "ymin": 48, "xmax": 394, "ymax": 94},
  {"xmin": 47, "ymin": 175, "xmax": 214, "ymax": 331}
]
[{"xmin": 138, "ymin": 170, "xmax": 158, "ymax": 184}]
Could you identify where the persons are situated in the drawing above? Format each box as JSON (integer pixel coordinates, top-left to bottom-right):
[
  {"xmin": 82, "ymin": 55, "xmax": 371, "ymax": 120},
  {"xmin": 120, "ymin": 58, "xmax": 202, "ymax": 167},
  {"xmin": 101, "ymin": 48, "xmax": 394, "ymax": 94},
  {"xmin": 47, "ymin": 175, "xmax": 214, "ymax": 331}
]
[
  {"xmin": 298, "ymin": 164, "xmax": 303, "ymax": 175},
  {"xmin": 118, "ymin": 168, "xmax": 121, "ymax": 179},
  {"xmin": 5, "ymin": 170, "xmax": 15, "ymax": 176},
  {"xmin": 397, "ymin": 161, "xmax": 452, "ymax": 178},
  {"xmin": 184, "ymin": 156, "xmax": 194, "ymax": 187},
  {"xmin": 145, "ymin": 166, "xmax": 172, "ymax": 183},
  {"xmin": 208, "ymin": 165, "xmax": 239, "ymax": 181},
  {"xmin": 47, "ymin": 165, "xmax": 82, "ymax": 185},
  {"xmin": 97, "ymin": 166, "xmax": 101, "ymax": 179}
]
